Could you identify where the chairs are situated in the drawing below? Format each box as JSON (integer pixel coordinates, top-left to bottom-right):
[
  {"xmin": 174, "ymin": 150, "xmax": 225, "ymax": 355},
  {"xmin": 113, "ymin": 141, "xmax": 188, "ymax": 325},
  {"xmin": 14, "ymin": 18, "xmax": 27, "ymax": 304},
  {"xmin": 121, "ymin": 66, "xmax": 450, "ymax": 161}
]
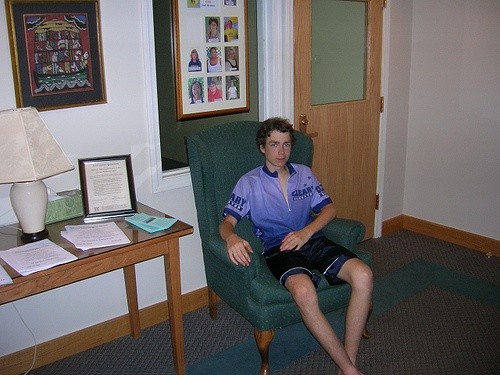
[{"xmin": 187, "ymin": 120, "xmax": 373, "ymax": 374}]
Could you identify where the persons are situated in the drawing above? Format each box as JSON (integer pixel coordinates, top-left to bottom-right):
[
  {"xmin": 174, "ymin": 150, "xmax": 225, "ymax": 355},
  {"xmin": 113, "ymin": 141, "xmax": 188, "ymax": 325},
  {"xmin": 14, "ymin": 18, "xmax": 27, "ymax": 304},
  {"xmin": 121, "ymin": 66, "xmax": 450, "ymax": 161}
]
[
  {"xmin": 206, "ymin": 17, "xmax": 221, "ymax": 42},
  {"xmin": 206, "ymin": 47, "xmax": 222, "ymax": 73},
  {"xmin": 218, "ymin": 117, "xmax": 375, "ymax": 375},
  {"xmin": 189, "ymin": 81, "xmax": 205, "ymax": 104},
  {"xmin": 225, "ymin": 47, "xmax": 239, "ymax": 71},
  {"xmin": 228, "ymin": 80, "xmax": 238, "ymax": 100},
  {"xmin": 208, "ymin": 81, "xmax": 222, "ymax": 102},
  {"xmin": 188, "ymin": 49, "xmax": 203, "ymax": 73},
  {"xmin": 224, "ymin": 20, "xmax": 238, "ymax": 42}
]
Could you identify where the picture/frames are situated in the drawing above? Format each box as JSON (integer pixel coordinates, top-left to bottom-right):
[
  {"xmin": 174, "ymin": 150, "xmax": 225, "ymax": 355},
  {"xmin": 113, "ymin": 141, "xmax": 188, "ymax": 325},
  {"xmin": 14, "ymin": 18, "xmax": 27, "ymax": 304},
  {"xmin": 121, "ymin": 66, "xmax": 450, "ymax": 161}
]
[
  {"xmin": 171, "ymin": 0, "xmax": 250, "ymax": 121},
  {"xmin": 5, "ymin": 0, "xmax": 107, "ymax": 112}
]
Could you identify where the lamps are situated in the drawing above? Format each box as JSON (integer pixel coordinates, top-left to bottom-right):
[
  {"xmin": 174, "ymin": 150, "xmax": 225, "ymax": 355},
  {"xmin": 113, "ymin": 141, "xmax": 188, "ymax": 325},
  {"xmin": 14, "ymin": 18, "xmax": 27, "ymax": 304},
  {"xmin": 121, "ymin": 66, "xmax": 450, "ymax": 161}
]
[{"xmin": 0, "ymin": 106, "xmax": 74, "ymax": 241}]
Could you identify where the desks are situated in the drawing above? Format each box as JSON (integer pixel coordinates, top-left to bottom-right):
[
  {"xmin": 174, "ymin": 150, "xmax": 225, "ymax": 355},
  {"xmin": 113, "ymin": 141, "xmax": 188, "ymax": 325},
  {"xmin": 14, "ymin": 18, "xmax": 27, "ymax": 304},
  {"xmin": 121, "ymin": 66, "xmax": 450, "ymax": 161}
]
[{"xmin": 0, "ymin": 201, "xmax": 195, "ymax": 375}]
